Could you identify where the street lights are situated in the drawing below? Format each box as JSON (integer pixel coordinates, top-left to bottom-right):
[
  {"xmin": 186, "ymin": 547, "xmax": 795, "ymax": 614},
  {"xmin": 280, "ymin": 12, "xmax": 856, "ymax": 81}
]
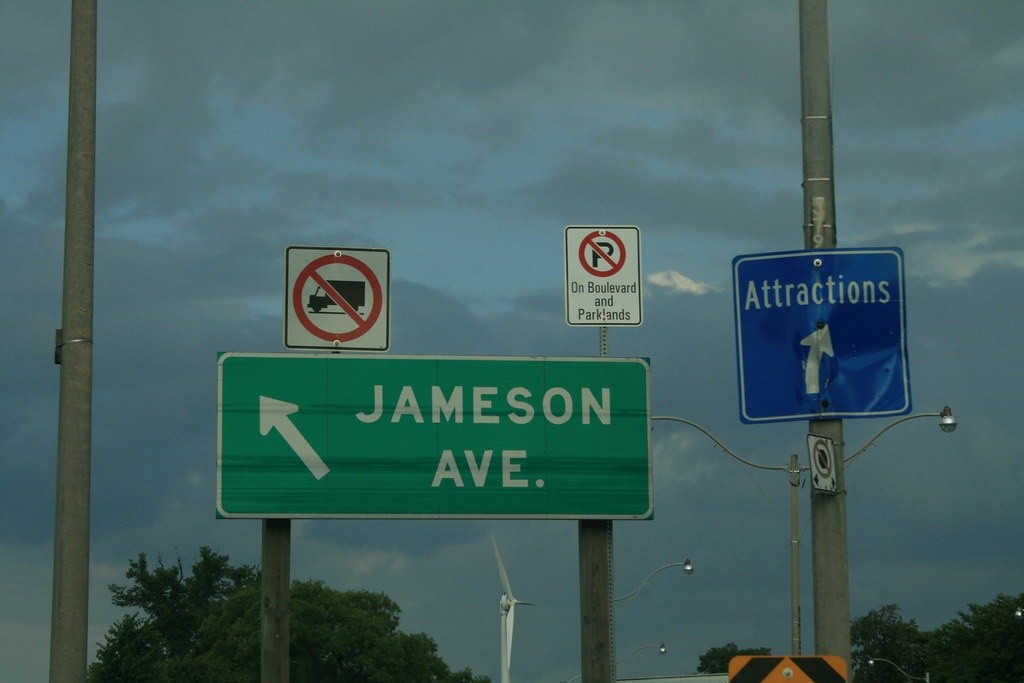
[{"xmin": 646, "ymin": 406, "xmax": 960, "ymax": 654}]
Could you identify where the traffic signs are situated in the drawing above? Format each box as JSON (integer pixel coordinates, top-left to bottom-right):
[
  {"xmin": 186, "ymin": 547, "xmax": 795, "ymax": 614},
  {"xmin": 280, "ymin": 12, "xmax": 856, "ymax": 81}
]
[{"xmin": 214, "ymin": 347, "xmax": 658, "ymax": 524}]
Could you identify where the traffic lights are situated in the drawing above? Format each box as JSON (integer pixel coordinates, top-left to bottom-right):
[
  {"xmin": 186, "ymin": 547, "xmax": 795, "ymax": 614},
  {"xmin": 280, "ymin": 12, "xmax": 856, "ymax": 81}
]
[{"xmin": 732, "ymin": 247, "xmax": 915, "ymax": 426}]
